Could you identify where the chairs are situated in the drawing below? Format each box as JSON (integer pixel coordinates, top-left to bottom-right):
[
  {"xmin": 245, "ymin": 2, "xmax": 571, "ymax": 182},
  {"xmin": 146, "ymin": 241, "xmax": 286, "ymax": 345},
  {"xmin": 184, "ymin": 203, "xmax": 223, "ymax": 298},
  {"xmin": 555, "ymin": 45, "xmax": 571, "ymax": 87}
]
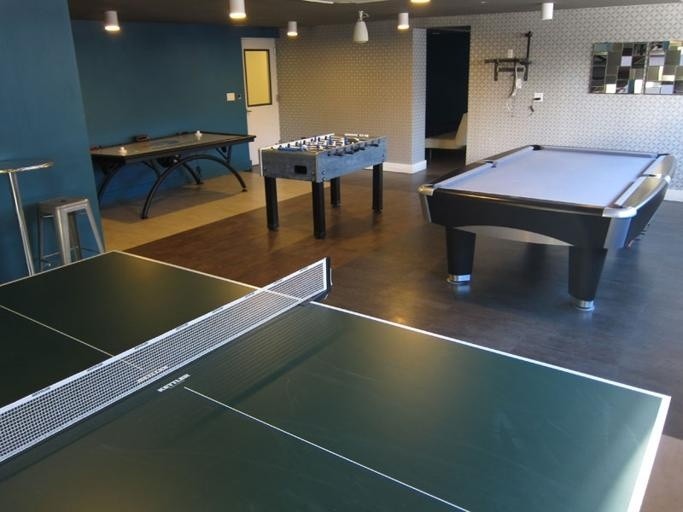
[{"xmin": 425, "ymin": 113, "xmax": 467, "ymax": 168}]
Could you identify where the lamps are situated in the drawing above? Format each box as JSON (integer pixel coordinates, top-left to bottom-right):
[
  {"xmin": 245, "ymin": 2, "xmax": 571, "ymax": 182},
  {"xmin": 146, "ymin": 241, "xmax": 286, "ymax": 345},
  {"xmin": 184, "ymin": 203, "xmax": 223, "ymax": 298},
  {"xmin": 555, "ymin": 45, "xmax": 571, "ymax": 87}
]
[
  {"xmin": 351, "ymin": 10, "xmax": 371, "ymax": 42},
  {"xmin": 540, "ymin": 2, "xmax": 553, "ymax": 23},
  {"xmin": 285, "ymin": 20, "xmax": 299, "ymax": 39},
  {"xmin": 103, "ymin": 8, "xmax": 121, "ymax": 33},
  {"xmin": 395, "ymin": 11, "xmax": 412, "ymax": 31},
  {"xmin": 227, "ymin": 1, "xmax": 248, "ymax": 22}
]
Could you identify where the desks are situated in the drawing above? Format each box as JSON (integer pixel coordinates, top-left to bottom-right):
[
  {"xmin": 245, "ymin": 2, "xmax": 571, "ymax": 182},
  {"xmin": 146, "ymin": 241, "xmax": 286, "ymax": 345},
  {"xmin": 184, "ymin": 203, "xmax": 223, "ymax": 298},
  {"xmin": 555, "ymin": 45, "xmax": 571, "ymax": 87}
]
[
  {"xmin": 0, "ymin": 159, "xmax": 56, "ymax": 276},
  {"xmin": 415, "ymin": 145, "xmax": 676, "ymax": 312},
  {"xmin": 89, "ymin": 131, "xmax": 256, "ymax": 220},
  {"xmin": 1, "ymin": 250, "xmax": 672, "ymax": 512}
]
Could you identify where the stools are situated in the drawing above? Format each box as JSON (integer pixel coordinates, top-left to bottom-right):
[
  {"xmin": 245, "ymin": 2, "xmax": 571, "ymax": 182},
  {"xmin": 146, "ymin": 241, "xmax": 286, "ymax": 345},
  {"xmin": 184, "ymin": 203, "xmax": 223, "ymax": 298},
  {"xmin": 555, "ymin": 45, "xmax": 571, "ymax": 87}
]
[{"xmin": 37, "ymin": 197, "xmax": 105, "ymax": 272}]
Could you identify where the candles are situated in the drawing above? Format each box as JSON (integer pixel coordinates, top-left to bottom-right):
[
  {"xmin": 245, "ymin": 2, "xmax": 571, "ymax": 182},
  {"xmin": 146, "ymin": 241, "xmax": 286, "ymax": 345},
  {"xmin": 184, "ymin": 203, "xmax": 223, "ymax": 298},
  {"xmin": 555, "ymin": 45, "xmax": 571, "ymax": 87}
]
[{"xmin": 508, "ymin": 49, "xmax": 513, "ymax": 58}]
